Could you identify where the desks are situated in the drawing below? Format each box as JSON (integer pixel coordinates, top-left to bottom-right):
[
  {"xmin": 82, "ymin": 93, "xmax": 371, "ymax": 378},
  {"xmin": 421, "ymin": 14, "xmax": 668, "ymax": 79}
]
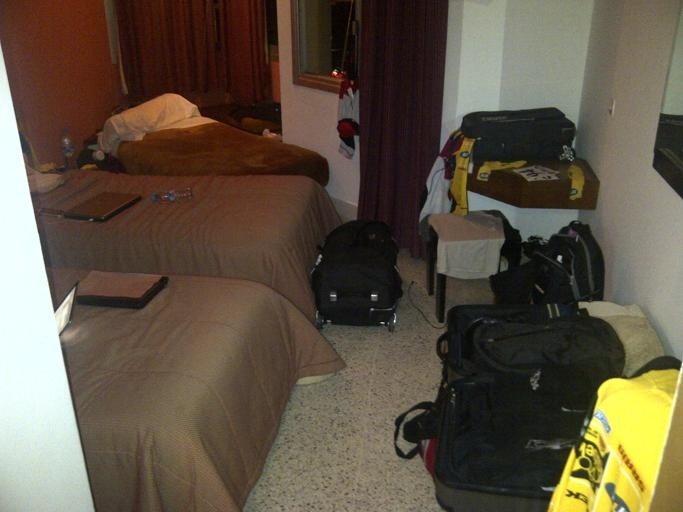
[{"xmin": 425, "ymin": 156, "xmax": 598, "ymax": 263}]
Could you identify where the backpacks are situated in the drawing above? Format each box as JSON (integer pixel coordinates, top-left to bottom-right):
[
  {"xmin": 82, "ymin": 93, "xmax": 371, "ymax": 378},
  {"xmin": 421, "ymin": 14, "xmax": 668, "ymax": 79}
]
[
  {"xmin": 390, "ymin": 301, "xmax": 628, "ymax": 512},
  {"xmin": 309, "ymin": 218, "xmax": 404, "ymax": 329},
  {"xmin": 461, "ymin": 105, "xmax": 577, "ymax": 164},
  {"xmin": 525, "ymin": 220, "xmax": 605, "ymax": 305}
]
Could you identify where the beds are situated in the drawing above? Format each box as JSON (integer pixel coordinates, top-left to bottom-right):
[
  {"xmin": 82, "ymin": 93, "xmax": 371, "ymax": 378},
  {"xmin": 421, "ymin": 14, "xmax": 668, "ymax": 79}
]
[
  {"xmin": 50, "ymin": 267, "xmax": 345, "ymax": 512},
  {"xmin": 87, "ymin": 92, "xmax": 332, "ymax": 186},
  {"xmin": 29, "ymin": 163, "xmax": 341, "ymax": 331}
]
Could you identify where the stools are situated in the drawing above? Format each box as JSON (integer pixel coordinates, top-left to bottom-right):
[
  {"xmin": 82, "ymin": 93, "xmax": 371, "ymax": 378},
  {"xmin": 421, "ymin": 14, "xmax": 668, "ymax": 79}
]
[{"xmin": 419, "ymin": 207, "xmax": 524, "ymax": 323}]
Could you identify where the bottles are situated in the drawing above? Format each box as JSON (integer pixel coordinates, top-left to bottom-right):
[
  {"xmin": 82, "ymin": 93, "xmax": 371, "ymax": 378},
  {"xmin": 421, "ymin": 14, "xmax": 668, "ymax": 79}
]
[
  {"xmin": 60, "ymin": 127, "xmax": 76, "ymax": 170},
  {"xmin": 149, "ymin": 186, "xmax": 194, "ymax": 203}
]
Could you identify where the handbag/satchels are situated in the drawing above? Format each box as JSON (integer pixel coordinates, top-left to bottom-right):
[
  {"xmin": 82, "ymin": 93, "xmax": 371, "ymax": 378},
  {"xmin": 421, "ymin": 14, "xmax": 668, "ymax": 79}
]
[{"xmin": 546, "ymin": 368, "xmax": 680, "ymax": 511}]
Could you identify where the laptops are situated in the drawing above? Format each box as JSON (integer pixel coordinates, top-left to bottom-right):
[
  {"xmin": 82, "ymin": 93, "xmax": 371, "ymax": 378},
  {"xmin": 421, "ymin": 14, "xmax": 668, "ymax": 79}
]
[{"xmin": 54, "ymin": 282, "xmax": 78, "ymax": 335}]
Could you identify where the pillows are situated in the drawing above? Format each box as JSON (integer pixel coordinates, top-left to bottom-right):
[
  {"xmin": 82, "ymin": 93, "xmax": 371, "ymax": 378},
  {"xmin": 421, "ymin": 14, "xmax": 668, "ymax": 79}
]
[
  {"xmin": 30, "ymin": 172, "xmax": 63, "ymax": 193},
  {"xmin": 97, "ymin": 93, "xmax": 200, "ymax": 152}
]
